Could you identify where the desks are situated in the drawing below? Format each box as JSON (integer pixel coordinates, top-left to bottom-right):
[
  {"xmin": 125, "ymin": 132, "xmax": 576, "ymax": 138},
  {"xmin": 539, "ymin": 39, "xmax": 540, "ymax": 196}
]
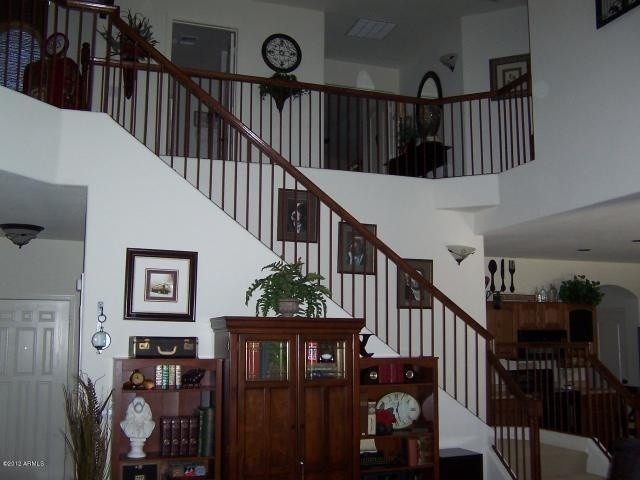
[{"xmin": 384, "ymin": 138, "xmax": 454, "ymax": 177}]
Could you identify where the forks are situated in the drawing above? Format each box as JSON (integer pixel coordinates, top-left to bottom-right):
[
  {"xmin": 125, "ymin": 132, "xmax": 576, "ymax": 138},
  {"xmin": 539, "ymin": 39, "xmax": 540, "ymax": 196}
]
[{"xmin": 509, "ymin": 259, "xmax": 515, "ymax": 292}]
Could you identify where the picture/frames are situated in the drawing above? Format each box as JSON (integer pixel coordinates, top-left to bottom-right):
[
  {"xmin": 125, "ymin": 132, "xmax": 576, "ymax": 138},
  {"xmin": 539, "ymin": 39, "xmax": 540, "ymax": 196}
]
[
  {"xmin": 124, "ymin": 248, "xmax": 198, "ymax": 322},
  {"xmin": 489, "ymin": 53, "xmax": 531, "ymax": 101},
  {"xmin": 337, "ymin": 219, "xmax": 379, "ymax": 276},
  {"xmin": 595, "ymin": 0, "xmax": 640, "ymax": 30},
  {"xmin": 396, "ymin": 257, "xmax": 435, "ymax": 309},
  {"xmin": 277, "ymin": 188, "xmax": 322, "ymax": 245}
]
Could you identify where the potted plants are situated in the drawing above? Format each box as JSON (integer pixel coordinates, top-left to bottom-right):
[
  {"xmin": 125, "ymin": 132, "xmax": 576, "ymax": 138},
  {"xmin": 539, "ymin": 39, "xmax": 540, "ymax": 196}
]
[
  {"xmin": 560, "ymin": 272, "xmax": 605, "ymax": 305},
  {"xmin": 101, "ymin": 5, "xmax": 161, "ymax": 98},
  {"xmin": 244, "ymin": 255, "xmax": 332, "ymax": 320}
]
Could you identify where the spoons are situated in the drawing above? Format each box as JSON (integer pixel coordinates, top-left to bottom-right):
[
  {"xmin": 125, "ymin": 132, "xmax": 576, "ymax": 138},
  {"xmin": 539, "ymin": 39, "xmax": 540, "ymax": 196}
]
[{"xmin": 488, "ymin": 259, "xmax": 498, "ymax": 293}]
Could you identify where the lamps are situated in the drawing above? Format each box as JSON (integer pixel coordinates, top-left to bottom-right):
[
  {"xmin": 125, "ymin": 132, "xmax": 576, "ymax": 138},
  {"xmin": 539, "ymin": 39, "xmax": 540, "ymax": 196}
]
[
  {"xmin": 440, "ymin": 52, "xmax": 458, "ymax": 72},
  {"xmin": 260, "ymin": 70, "xmax": 310, "ymax": 110},
  {"xmin": 1, "ymin": 222, "xmax": 47, "ymax": 251},
  {"xmin": 445, "ymin": 243, "xmax": 476, "ymax": 265}
]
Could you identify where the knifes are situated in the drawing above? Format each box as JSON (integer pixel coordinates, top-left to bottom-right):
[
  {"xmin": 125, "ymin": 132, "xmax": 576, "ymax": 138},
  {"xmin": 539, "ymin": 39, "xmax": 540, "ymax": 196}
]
[{"xmin": 500, "ymin": 259, "xmax": 507, "ymax": 291}]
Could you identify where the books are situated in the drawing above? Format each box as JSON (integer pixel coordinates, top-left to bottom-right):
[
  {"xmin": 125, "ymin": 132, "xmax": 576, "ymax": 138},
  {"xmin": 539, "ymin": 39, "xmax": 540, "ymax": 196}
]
[{"xmin": 156, "ymin": 363, "xmax": 215, "ymax": 457}]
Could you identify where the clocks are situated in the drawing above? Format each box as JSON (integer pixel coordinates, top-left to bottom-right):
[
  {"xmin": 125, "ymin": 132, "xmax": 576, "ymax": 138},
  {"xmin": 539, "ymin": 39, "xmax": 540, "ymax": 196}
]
[{"xmin": 376, "ymin": 390, "xmax": 423, "ymax": 431}]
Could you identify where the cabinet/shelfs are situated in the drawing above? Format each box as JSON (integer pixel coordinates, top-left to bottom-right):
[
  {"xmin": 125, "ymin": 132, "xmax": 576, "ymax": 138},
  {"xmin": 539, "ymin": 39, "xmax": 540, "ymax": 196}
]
[
  {"xmin": 514, "ymin": 303, "xmax": 567, "ymax": 331},
  {"xmin": 210, "ymin": 314, "xmax": 367, "ymax": 480},
  {"xmin": 358, "ymin": 356, "xmax": 441, "ymax": 479},
  {"xmin": 487, "ymin": 300, "xmax": 518, "ymax": 358},
  {"xmin": 558, "ymin": 304, "xmax": 599, "ymax": 369},
  {"xmin": 110, "ymin": 356, "xmax": 226, "ymax": 480}
]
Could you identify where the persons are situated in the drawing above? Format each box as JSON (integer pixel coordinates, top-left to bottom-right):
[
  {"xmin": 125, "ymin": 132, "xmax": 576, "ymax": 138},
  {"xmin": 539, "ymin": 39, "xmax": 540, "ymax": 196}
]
[
  {"xmin": 407, "ymin": 270, "xmax": 424, "ymax": 301},
  {"xmin": 345, "ymin": 237, "xmax": 364, "ymax": 265}
]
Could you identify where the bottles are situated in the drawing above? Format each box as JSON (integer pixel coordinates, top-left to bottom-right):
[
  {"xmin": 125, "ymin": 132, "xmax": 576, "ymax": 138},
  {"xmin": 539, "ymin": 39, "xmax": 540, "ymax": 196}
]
[{"xmin": 535, "ymin": 284, "xmax": 558, "ymax": 302}]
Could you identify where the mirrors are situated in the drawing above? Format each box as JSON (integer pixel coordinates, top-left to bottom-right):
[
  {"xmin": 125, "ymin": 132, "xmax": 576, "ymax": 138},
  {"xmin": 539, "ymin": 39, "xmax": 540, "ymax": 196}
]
[{"xmin": 415, "ymin": 70, "xmax": 443, "ymax": 137}]
